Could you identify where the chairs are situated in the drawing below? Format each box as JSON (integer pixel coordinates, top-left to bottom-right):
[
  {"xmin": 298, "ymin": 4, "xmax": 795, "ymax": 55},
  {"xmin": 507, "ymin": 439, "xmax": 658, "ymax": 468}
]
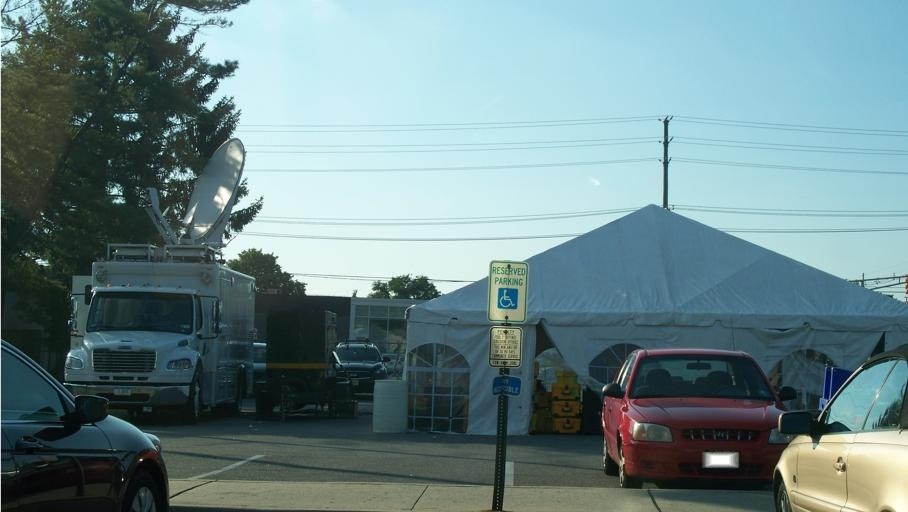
[{"xmin": 632, "ymin": 368, "xmax": 740, "ymax": 394}]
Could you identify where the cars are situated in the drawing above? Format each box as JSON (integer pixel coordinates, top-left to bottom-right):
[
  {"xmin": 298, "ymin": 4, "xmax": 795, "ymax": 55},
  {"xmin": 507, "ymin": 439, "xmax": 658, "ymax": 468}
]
[
  {"xmin": 251, "ymin": 307, "xmax": 404, "ymax": 418},
  {"xmin": 598, "ymin": 347, "xmax": 795, "ymax": 493},
  {"xmin": 1, "ymin": 338, "xmax": 170, "ymax": 509},
  {"xmin": 766, "ymin": 340, "xmax": 907, "ymax": 512}
]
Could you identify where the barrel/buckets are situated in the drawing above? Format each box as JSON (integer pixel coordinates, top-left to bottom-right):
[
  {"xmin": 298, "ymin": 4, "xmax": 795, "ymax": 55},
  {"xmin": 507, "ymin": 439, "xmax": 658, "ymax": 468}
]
[{"xmin": 372, "ymin": 380, "xmax": 408, "ymax": 434}]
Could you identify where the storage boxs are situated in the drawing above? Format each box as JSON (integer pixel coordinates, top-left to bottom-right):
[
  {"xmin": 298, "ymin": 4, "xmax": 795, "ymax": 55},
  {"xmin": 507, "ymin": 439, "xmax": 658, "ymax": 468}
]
[{"xmin": 529, "ymin": 370, "xmax": 582, "ymax": 434}]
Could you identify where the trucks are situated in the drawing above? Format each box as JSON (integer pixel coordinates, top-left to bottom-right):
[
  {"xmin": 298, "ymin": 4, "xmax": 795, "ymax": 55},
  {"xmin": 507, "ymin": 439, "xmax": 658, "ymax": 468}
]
[{"xmin": 61, "ymin": 135, "xmax": 259, "ymax": 424}]
